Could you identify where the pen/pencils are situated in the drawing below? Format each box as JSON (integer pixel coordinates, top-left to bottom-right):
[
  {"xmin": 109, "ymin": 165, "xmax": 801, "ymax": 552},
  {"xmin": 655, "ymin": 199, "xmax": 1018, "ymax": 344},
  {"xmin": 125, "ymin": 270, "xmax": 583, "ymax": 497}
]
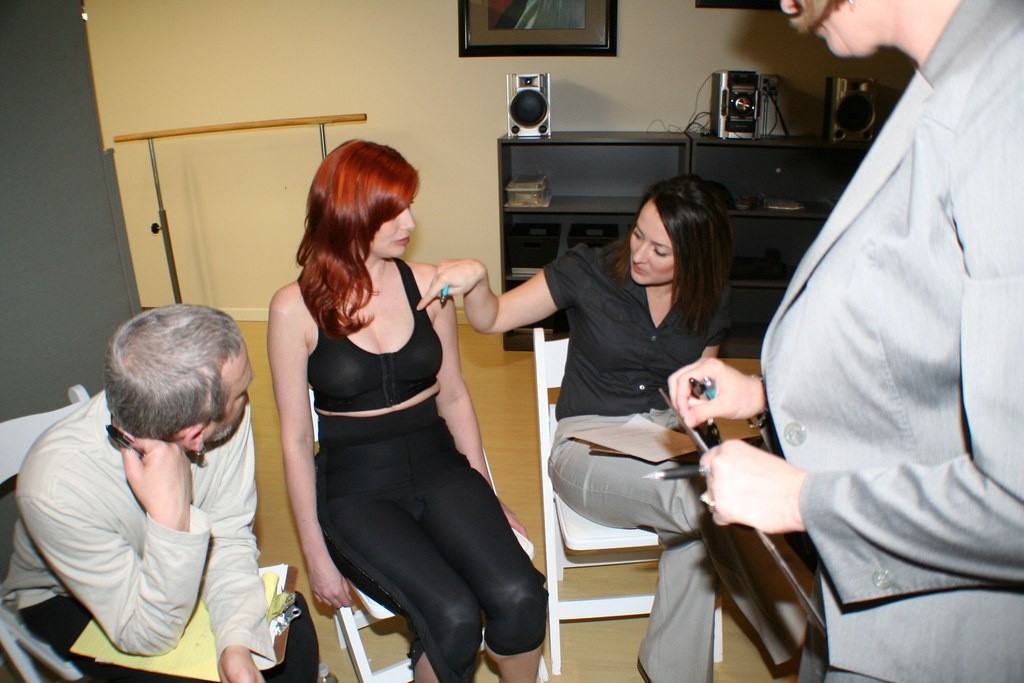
[
  {"xmin": 441, "ymin": 286, "xmax": 449, "ymax": 303},
  {"xmin": 106, "ymin": 425, "xmax": 144, "ymax": 458},
  {"xmin": 704, "ymin": 376, "xmax": 716, "ymax": 400},
  {"xmin": 640, "ymin": 466, "xmax": 708, "ymax": 481},
  {"xmin": 689, "ymin": 378, "xmax": 709, "ymax": 400}
]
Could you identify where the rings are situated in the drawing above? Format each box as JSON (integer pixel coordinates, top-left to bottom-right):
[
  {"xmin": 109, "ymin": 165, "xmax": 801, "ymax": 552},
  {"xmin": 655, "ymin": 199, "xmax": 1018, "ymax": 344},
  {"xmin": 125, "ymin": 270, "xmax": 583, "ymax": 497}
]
[
  {"xmin": 699, "ymin": 491, "xmax": 715, "ymax": 508},
  {"xmin": 697, "ymin": 466, "xmax": 710, "ymax": 473}
]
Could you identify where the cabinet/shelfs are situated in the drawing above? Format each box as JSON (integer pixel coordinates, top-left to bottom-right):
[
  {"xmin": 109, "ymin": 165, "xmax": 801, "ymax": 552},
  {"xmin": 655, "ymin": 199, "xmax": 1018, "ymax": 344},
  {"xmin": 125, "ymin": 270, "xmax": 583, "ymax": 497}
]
[
  {"xmin": 497, "ymin": 133, "xmax": 691, "ymax": 351},
  {"xmin": 688, "ymin": 131, "xmax": 873, "ymax": 358}
]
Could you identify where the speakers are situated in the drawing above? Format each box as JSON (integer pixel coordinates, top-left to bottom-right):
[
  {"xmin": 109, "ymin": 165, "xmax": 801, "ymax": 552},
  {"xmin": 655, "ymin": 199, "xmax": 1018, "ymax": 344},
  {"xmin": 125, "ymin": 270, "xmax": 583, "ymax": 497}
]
[
  {"xmin": 507, "ymin": 72, "xmax": 551, "ymax": 138},
  {"xmin": 823, "ymin": 76, "xmax": 875, "ymax": 142}
]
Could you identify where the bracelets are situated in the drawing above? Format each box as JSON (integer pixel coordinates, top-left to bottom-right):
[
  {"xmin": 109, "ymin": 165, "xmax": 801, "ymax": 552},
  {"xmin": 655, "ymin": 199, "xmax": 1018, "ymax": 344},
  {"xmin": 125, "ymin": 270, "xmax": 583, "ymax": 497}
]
[{"xmin": 746, "ymin": 372, "xmax": 767, "ymax": 428}]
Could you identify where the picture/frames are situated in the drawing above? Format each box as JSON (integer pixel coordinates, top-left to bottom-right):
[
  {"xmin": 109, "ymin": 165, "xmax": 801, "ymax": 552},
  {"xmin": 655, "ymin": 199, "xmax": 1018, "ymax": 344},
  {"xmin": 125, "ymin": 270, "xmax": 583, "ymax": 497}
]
[{"xmin": 457, "ymin": 0, "xmax": 618, "ymax": 57}]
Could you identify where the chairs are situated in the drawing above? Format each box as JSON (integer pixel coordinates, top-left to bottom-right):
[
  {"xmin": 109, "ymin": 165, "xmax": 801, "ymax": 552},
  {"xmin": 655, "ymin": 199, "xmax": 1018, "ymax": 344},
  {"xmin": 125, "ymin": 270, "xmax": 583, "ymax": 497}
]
[
  {"xmin": 0, "ymin": 384, "xmax": 90, "ymax": 683},
  {"xmin": 533, "ymin": 327, "xmax": 723, "ymax": 674},
  {"xmin": 309, "ymin": 388, "xmax": 549, "ymax": 683}
]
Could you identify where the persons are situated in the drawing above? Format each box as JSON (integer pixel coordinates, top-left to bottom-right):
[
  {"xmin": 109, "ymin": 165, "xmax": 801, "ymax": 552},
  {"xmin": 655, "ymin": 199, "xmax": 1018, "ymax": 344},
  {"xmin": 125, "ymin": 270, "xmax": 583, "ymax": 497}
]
[
  {"xmin": 267, "ymin": 140, "xmax": 549, "ymax": 683},
  {"xmin": 416, "ymin": 172, "xmax": 808, "ymax": 683},
  {"xmin": 0, "ymin": 303, "xmax": 324, "ymax": 683},
  {"xmin": 669, "ymin": 0, "xmax": 1023, "ymax": 683}
]
